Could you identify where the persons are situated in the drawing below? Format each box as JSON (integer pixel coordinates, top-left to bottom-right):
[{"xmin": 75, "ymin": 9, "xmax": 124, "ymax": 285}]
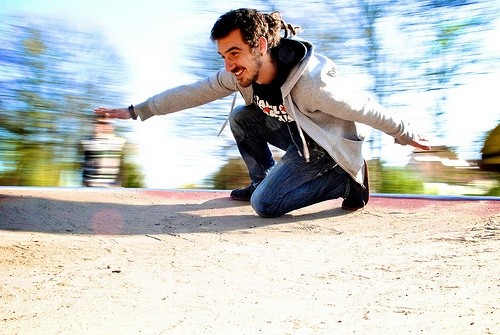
[
  {"xmin": 92, "ymin": 7, "xmax": 432, "ymax": 221},
  {"xmin": 79, "ymin": 117, "xmax": 127, "ymax": 190}
]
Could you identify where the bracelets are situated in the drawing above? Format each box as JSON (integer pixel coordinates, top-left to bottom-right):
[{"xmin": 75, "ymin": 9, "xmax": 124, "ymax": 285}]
[{"xmin": 128, "ymin": 104, "xmax": 137, "ymax": 120}]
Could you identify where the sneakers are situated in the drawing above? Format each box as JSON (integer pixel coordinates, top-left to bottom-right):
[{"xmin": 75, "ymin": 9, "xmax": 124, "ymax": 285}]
[
  {"xmin": 342, "ymin": 159, "xmax": 370, "ymax": 211},
  {"xmin": 230, "ymin": 182, "xmax": 258, "ymax": 201}
]
